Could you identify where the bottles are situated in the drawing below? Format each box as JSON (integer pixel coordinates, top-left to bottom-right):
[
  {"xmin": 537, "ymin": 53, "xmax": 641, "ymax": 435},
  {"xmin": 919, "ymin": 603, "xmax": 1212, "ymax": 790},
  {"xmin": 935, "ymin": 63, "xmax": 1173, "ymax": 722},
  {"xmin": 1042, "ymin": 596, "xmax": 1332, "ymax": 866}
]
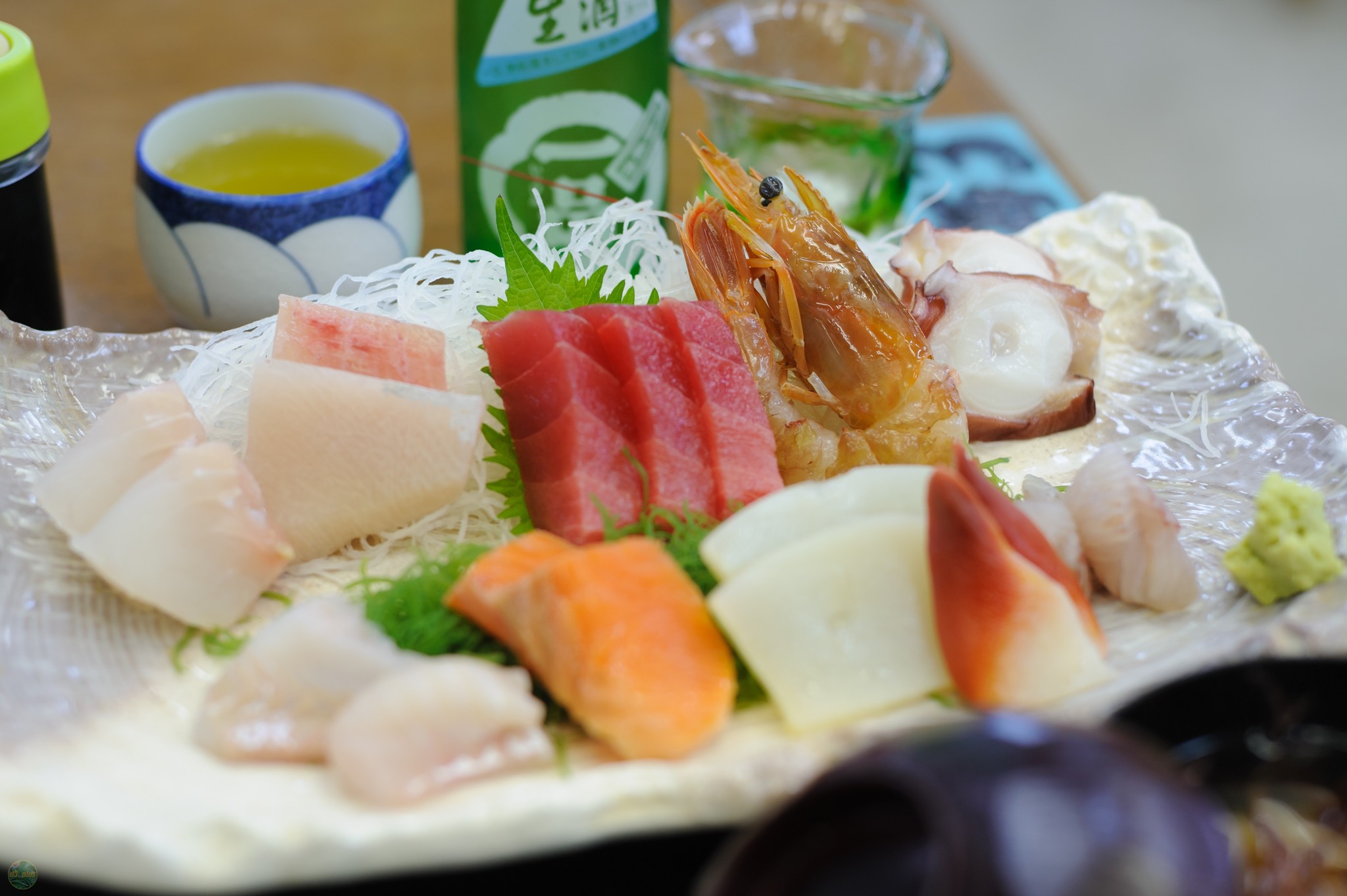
[{"xmin": 1, "ymin": 21, "xmax": 68, "ymax": 334}]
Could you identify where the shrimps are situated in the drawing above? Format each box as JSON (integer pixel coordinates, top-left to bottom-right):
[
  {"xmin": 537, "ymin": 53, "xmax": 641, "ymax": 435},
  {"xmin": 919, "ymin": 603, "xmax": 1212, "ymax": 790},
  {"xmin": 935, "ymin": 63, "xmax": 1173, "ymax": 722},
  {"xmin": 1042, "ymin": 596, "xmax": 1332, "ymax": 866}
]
[{"xmin": 674, "ymin": 128, "xmax": 982, "ymax": 478}]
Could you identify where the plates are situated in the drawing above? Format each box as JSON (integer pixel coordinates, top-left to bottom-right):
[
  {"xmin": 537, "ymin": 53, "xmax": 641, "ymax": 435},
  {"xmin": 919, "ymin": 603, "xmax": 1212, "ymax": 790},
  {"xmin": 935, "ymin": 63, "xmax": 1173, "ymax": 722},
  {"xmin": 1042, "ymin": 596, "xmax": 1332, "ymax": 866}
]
[{"xmin": 2, "ymin": 195, "xmax": 1346, "ymax": 894}]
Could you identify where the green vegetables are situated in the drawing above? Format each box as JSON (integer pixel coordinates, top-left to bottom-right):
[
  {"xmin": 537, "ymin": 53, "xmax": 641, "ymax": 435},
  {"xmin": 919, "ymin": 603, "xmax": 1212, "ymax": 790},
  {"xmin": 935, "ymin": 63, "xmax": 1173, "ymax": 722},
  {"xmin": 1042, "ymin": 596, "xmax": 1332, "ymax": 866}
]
[{"xmin": 167, "ymin": 191, "xmax": 765, "ymax": 753}]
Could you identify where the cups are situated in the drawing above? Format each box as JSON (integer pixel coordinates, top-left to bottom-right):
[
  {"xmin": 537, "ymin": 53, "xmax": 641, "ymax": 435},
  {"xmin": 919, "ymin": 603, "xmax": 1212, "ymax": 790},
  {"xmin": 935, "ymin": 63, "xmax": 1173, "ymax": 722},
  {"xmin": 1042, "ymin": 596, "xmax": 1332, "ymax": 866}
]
[
  {"xmin": 666, "ymin": 0, "xmax": 952, "ymax": 240},
  {"xmin": 130, "ymin": 83, "xmax": 424, "ymax": 337}
]
